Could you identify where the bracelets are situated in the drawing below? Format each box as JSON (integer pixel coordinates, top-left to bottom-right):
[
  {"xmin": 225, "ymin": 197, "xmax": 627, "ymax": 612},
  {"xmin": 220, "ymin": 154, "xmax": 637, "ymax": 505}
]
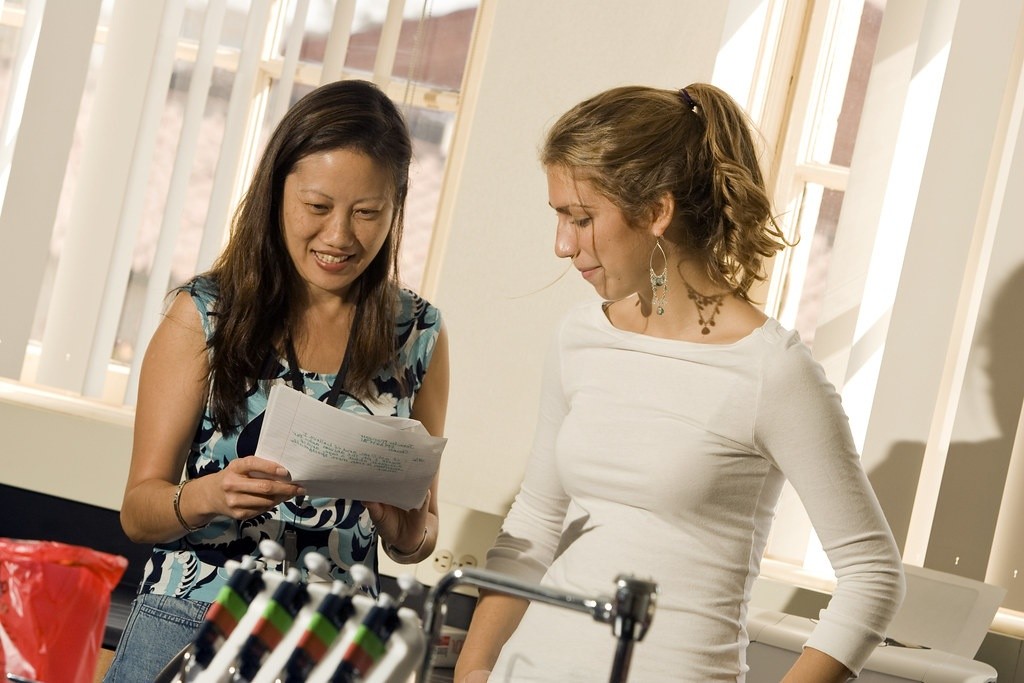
[
  {"xmin": 174, "ymin": 480, "xmax": 210, "ymax": 532},
  {"xmin": 387, "ymin": 527, "xmax": 428, "ymax": 558}
]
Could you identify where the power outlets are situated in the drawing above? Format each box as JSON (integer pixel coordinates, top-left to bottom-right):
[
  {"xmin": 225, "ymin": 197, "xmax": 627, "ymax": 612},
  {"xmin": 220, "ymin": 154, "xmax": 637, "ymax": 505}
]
[{"xmin": 429, "ymin": 550, "xmax": 477, "ymax": 575}]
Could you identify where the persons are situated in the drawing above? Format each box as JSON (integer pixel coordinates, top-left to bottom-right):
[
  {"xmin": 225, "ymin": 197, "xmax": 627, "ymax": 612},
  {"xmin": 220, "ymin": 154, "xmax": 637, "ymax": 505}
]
[
  {"xmin": 102, "ymin": 80, "xmax": 449, "ymax": 683},
  {"xmin": 451, "ymin": 83, "xmax": 906, "ymax": 683}
]
